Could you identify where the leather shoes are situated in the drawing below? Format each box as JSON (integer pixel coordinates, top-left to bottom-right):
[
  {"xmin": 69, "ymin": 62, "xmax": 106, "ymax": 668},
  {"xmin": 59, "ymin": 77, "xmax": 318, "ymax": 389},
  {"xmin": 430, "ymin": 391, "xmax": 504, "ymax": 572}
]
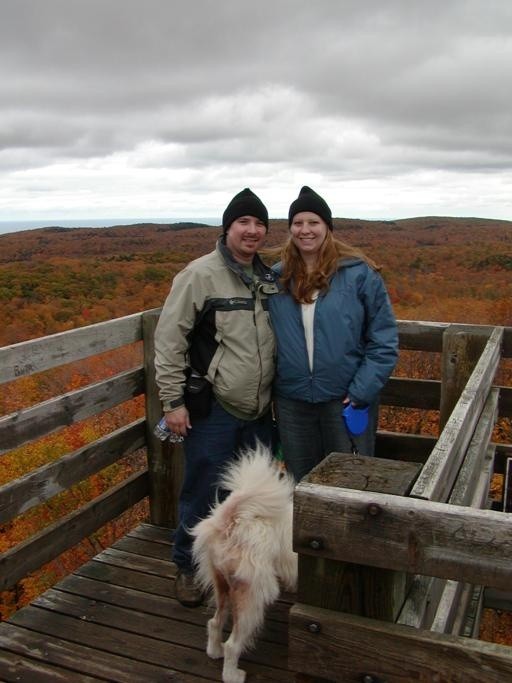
[{"xmin": 176, "ymin": 569, "xmax": 202, "ymax": 608}]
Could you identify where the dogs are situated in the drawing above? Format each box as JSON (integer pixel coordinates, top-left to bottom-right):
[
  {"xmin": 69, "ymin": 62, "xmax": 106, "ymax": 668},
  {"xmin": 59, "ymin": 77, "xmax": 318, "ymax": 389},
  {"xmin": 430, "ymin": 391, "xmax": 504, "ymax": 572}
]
[{"xmin": 181, "ymin": 432, "xmax": 299, "ymax": 683}]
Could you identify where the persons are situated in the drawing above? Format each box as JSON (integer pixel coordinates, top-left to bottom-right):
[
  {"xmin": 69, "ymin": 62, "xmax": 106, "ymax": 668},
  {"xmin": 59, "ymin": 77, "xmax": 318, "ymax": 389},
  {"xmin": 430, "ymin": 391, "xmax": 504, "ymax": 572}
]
[
  {"xmin": 153, "ymin": 188, "xmax": 276, "ymax": 608},
  {"xmin": 269, "ymin": 185, "xmax": 400, "ymax": 483}
]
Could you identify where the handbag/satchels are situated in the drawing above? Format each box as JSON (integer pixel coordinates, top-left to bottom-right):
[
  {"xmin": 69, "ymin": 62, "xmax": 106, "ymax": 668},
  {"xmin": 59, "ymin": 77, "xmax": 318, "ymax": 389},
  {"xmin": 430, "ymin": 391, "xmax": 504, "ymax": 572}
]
[{"xmin": 183, "ymin": 377, "xmax": 212, "ymax": 417}]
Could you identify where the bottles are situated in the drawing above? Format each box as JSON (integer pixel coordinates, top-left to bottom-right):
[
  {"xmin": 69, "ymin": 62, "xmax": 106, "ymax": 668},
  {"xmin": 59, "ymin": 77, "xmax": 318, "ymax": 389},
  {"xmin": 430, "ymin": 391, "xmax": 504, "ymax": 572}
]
[{"xmin": 152, "ymin": 414, "xmax": 185, "ymax": 443}]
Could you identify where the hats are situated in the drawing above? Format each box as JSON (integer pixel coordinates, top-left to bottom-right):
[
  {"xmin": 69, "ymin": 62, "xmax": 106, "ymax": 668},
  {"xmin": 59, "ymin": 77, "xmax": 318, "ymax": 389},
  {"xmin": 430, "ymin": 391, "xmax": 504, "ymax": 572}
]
[
  {"xmin": 289, "ymin": 186, "xmax": 333, "ymax": 232},
  {"xmin": 223, "ymin": 188, "xmax": 268, "ymax": 234}
]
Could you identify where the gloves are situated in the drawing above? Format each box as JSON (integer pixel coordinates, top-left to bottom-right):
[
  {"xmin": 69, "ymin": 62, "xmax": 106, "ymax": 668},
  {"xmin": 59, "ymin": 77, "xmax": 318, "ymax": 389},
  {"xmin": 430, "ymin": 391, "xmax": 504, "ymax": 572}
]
[{"xmin": 342, "ymin": 402, "xmax": 369, "ymax": 435}]
